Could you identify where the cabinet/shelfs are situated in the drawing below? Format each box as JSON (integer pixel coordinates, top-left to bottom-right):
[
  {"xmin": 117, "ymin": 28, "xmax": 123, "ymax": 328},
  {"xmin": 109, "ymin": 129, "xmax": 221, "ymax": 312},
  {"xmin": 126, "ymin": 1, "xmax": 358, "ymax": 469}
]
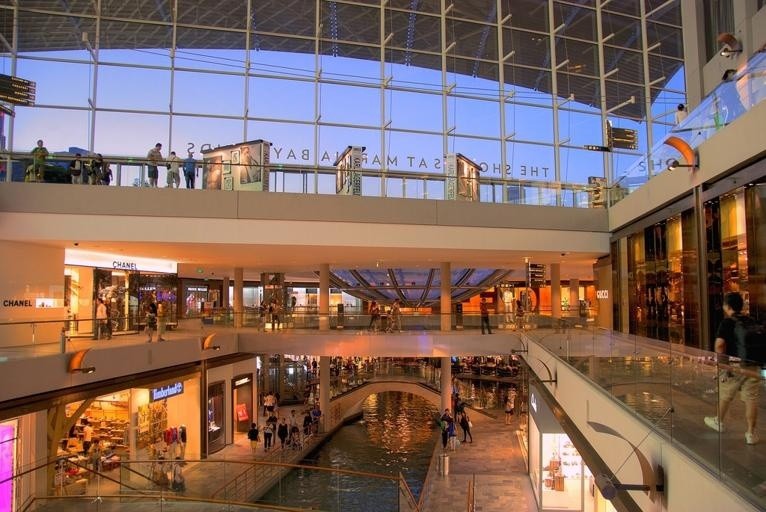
[{"xmin": 52, "ymin": 415, "xmax": 130, "ymax": 497}]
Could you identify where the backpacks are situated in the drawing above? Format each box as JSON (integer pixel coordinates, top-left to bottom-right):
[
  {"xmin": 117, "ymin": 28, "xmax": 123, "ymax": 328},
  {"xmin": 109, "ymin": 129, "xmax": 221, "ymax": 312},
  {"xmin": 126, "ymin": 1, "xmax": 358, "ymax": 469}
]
[{"xmin": 726, "ymin": 315, "xmax": 765, "ymax": 368}]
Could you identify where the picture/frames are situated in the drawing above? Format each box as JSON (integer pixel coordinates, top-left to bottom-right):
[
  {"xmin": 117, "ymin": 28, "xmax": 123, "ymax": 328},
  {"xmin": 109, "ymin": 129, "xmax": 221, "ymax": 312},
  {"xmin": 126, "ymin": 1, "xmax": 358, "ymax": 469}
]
[
  {"xmin": 231, "ymin": 150, "xmax": 240, "ymax": 165},
  {"xmin": 222, "ymin": 161, "xmax": 231, "ymax": 174},
  {"xmin": 224, "ymin": 176, "xmax": 233, "ymax": 191}
]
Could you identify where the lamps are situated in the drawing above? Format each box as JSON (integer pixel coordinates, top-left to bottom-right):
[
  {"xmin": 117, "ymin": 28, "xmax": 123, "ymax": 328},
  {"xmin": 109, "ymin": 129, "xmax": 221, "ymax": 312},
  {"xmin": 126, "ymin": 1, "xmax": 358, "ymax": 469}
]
[
  {"xmin": 722, "ymin": 69, "xmax": 737, "ymax": 81},
  {"xmin": 74, "ymin": 367, "xmax": 95, "ymax": 374},
  {"xmin": 594, "ymin": 465, "xmax": 664, "ymax": 498},
  {"xmin": 206, "ymin": 346, "xmax": 221, "ymax": 351},
  {"xmin": 511, "ymin": 349, "xmax": 527, "ymax": 360},
  {"xmin": 720, "ymin": 48, "xmax": 742, "ymax": 57},
  {"xmin": 666, "ymin": 159, "xmax": 699, "ymax": 171},
  {"xmin": 527, "ymin": 378, "xmax": 557, "ymax": 388}
]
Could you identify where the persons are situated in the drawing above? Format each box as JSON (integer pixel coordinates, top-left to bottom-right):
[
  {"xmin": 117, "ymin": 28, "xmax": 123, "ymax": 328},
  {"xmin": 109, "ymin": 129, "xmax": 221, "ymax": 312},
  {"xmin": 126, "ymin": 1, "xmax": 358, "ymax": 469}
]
[
  {"xmin": 93, "ymin": 296, "xmax": 112, "ymax": 341},
  {"xmin": 676, "ymin": 103, "xmax": 688, "ymax": 125},
  {"xmin": 156, "ymin": 300, "xmax": 169, "ymax": 342},
  {"xmin": 172, "ymin": 456, "xmax": 187, "ymax": 497},
  {"xmin": 147, "ymin": 142, "xmax": 199, "ymax": 188},
  {"xmin": 516, "ymin": 299, "xmax": 523, "ymax": 332},
  {"xmin": 164, "ymin": 424, "xmax": 187, "ymax": 460},
  {"xmin": 29, "ymin": 140, "xmax": 49, "ymax": 183},
  {"xmin": 479, "ymin": 298, "xmax": 494, "ymax": 334},
  {"xmin": 142, "ymin": 303, "xmax": 157, "ymax": 342},
  {"xmin": 248, "ymin": 357, "xmax": 378, "ymax": 457},
  {"xmin": 262, "ymin": 295, "xmax": 296, "ymax": 332},
  {"xmin": 368, "ymin": 298, "xmax": 402, "ymax": 333},
  {"xmin": 69, "ymin": 153, "xmax": 113, "ymax": 185},
  {"xmin": 155, "ymin": 457, "xmax": 171, "ymax": 496},
  {"xmin": 720, "ymin": 68, "xmax": 746, "ymax": 127},
  {"xmin": 503, "ymin": 396, "xmax": 513, "ymax": 425},
  {"xmin": 704, "ymin": 292, "xmax": 766, "ymax": 444},
  {"xmin": 451, "ymin": 354, "xmax": 520, "ymax": 377},
  {"xmin": 440, "ymin": 381, "xmax": 473, "ymax": 448},
  {"xmin": 69, "ymin": 420, "xmax": 104, "ymax": 477}
]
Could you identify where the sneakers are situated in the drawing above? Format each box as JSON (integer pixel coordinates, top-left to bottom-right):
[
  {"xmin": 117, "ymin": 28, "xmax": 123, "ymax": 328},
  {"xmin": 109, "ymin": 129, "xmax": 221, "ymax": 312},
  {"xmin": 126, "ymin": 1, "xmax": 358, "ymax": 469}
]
[
  {"xmin": 704, "ymin": 416, "xmax": 726, "ymax": 432},
  {"xmin": 744, "ymin": 431, "xmax": 761, "ymax": 444}
]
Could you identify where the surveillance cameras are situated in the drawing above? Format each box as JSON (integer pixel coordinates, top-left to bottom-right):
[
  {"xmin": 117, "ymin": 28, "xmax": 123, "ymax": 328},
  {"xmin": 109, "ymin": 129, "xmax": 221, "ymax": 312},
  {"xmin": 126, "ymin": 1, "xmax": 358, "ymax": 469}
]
[{"xmin": 72, "ymin": 241, "xmax": 79, "ymax": 247}]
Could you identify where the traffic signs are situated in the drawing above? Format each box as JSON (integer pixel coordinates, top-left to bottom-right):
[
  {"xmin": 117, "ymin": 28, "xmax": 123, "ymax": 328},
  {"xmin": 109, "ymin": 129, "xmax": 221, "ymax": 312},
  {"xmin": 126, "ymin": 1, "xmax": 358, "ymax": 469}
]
[
  {"xmin": 525, "ymin": 262, "xmax": 546, "ymax": 288},
  {"xmin": 582, "ymin": 125, "xmax": 638, "ymax": 151},
  {"xmin": 0, "ymin": 73, "xmax": 36, "ymax": 117}
]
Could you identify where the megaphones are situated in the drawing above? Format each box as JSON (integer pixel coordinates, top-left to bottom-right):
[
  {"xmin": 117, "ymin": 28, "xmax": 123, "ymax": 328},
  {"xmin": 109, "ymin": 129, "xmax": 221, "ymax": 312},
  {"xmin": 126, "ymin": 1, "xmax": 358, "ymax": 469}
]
[{"xmin": 666, "ymin": 157, "xmax": 680, "ymax": 171}]
